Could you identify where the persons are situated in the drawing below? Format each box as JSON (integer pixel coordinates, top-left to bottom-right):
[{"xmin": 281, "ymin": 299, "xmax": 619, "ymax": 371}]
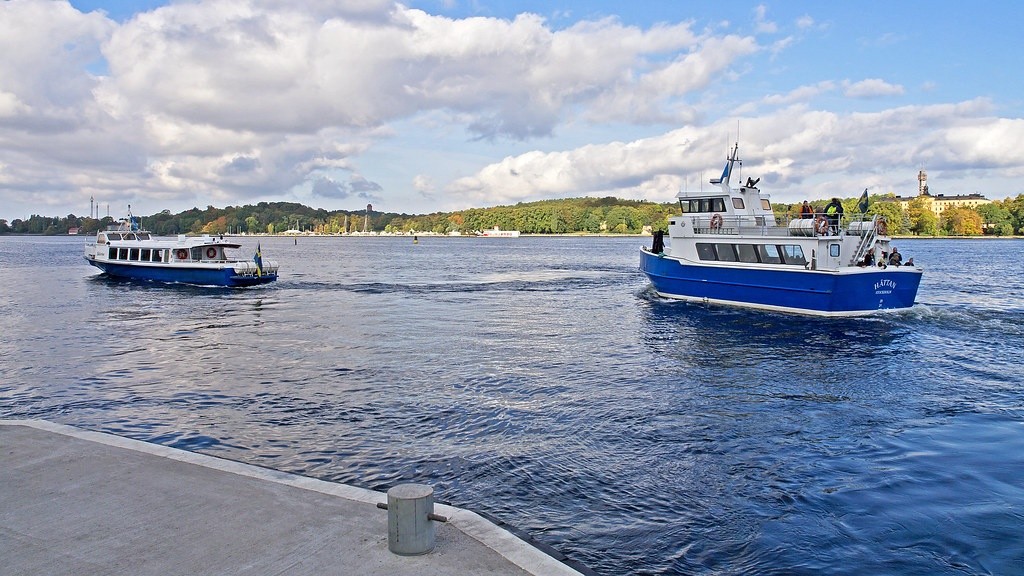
[
  {"xmin": 858, "ymin": 248, "xmax": 876, "ymax": 266},
  {"xmin": 798, "ymin": 200, "xmax": 815, "ymax": 219},
  {"xmin": 878, "ymin": 247, "xmax": 902, "ymax": 267},
  {"xmin": 830, "ymin": 198, "xmax": 844, "ymax": 235},
  {"xmin": 904, "ymin": 257, "xmax": 914, "ymax": 266}
]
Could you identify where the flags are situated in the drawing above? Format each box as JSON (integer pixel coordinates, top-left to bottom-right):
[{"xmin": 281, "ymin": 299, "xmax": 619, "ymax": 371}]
[
  {"xmin": 253, "ymin": 243, "xmax": 262, "ymax": 277},
  {"xmin": 721, "ymin": 164, "xmax": 729, "ymax": 185},
  {"xmin": 130, "ymin": 215, "xmax": 138, "ymax": 231}
]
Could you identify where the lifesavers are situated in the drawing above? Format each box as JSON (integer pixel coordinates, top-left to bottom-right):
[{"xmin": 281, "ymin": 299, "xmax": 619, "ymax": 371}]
[
  {"xmin": 876, "ymin": 220, "xmax": 887, "ymax": 235},
  {"xmin": 206, "ymin": 248, "xmax": 217, "ymax": 258},
  {"xmin": 177, "ymin": 249, "xmax": 188, "ymax": 259},
  {"xmin": 815, "ymin": 217, "xmax": 829, "ymax": 233},
  {"xmin": 710, "ymin": 214, "xmax": 723, "ymax": 229}
]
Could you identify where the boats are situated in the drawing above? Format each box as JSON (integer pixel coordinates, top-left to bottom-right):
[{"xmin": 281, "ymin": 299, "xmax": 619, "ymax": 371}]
[
  {"xmin": 82, "ymin": 202, "xmax": 280, "ymax": 289},
  {"xmin": 637, "ymin": 119, "xmax": 922, "ymax": 320}
]
